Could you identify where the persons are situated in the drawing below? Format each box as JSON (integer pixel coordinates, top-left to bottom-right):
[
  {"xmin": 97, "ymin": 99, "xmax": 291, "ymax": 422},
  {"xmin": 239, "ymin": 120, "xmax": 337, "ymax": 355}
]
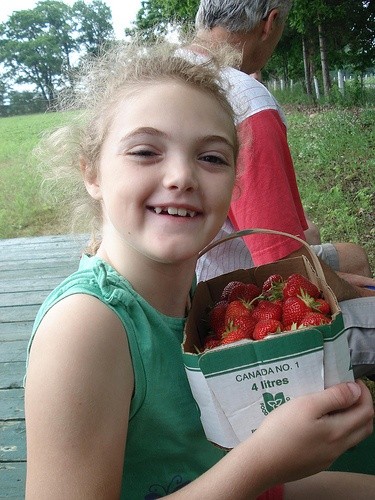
[
  {"xmin": 171, "ymin": 0, "xmax": 373, "ymax": 381},
  {"xmin": 21, "ymin": 20, "xmax": 374, "ymax": 500}
]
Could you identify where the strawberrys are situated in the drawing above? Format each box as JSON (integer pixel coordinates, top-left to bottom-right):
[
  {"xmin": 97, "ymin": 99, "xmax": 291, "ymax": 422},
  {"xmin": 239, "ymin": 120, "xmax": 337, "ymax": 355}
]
[{"xmin": 192, "ymin": 272, "xmax": 333, "ymax": 354}]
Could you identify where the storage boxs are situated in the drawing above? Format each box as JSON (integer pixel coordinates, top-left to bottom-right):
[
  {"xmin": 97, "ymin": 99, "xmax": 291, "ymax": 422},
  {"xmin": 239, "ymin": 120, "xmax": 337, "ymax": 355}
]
[{"xmin": 180, "ymin": 254, "xmax": 356, "ymax": 452}]
